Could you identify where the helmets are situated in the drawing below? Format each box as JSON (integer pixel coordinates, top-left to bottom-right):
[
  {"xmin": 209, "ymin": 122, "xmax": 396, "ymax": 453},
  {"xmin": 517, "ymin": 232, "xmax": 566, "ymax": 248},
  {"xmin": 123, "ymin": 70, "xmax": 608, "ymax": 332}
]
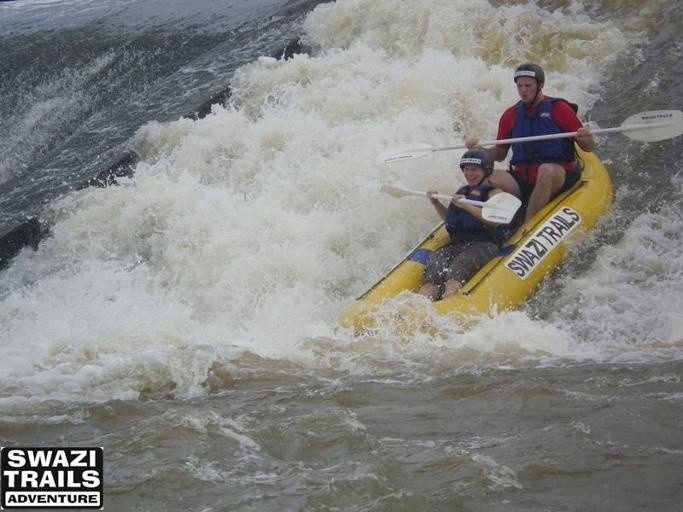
[
  {"xmin": 460, "ymin": 150, "xmax": 494, "ymax": 170},
  {"xmin": 514, "ymin": 63, "xmax": 544, "ymax": 83}
]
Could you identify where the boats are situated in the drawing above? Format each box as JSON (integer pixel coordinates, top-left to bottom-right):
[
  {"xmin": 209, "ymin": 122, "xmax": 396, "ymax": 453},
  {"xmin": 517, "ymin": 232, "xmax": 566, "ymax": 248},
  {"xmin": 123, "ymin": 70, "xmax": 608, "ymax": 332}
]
[{"xmin": 333, "ymin": 139, "xmax": 616, "ymax": 344}]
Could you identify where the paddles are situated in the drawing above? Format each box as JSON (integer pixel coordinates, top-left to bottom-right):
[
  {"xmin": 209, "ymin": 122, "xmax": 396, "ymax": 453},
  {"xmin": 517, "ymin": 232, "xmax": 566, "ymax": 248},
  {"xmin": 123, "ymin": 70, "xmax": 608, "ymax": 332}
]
[
  {"xmin": 381, "ymin": 184, "xmax": 523, "ymax": 225},
  {"xmin": 375, "ymin": 109, "xmax": 683, "ymax": 166}
]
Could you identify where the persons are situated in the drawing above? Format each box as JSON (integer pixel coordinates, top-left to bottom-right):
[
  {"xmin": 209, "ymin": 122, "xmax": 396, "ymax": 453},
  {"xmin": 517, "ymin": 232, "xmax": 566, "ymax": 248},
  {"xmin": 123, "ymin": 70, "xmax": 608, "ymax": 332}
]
[
  {"xmin": 461, "ymin": 61, "xmax": 595, "ymax": 224},
  {"xmin": 416, "ymin": 145, "xmax": 507, "ymax": 301}
]
